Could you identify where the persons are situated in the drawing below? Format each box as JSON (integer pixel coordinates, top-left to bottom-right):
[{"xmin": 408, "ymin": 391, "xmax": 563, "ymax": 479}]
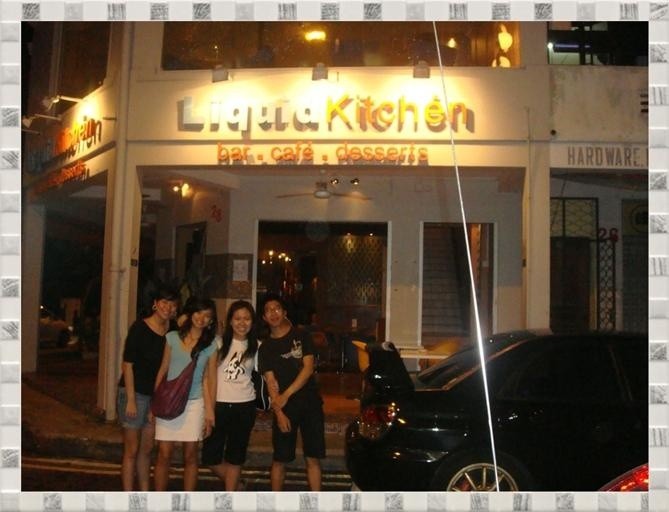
[
  {"xmin": 115, "ymin": 286, "xmax": 182, "ymax": 491},
  {"xmin": 201, "ymin": 299, "xmax": 281, "ymax": 492},
  {"xmin": 148, "ymin": 298, "xmax": 220, "ymax": 493},
  {"xmin": 256, "ymin": 296, "xmax": 328, "ymax": 491},
  {"xmin": 176, "ymin": 296, "xmax": 199, "ymax": 328}
]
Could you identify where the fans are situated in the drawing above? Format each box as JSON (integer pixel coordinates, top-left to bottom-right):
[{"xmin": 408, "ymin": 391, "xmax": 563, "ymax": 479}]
[{"xmin": 274, "ymin": 181, "xmax": 374, "ymax": 204}]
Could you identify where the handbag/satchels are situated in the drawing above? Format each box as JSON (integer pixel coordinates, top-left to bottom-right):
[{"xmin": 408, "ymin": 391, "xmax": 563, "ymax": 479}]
[{"xmin": 150, "ymin": 360, "xmax": 196, "ymax": 420}]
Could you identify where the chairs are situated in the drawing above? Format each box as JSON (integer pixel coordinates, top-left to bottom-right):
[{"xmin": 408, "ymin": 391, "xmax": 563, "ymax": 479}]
[
  {"xmin": 351, "ymin": 339, "xmax": 369, "ymax": 395},
  {"xmin": 308, "ymin": 332, "xmax": 330, "ymax": 373},
  {"xmin": 419, "ymin": 337, "xmax": 460, "ymax": 371}
]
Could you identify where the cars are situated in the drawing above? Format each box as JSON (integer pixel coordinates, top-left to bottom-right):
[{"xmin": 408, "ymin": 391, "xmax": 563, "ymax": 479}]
[{"xmin": 344, "ymin": 327, "xmax": 648, "ymax": 492}]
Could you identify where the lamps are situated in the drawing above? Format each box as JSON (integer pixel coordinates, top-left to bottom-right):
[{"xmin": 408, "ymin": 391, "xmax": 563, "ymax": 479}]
[
  {"xmin": 21, "ymin": 112, "xmax": 62, "ymax": 129},
  {"xmin": 311, "ymin": 61, "xmax": 328, "ymax": 79},
  {"xmin": 412, "ymin": 60, "xmax": 430, "ymax": 79},
  {"xmin": 209, "ymin": 44, "xmax": 229, "ymax": 83},
  {"xmin": 41, "ymin": 95, "xmax": 83, "ymax": 109}
]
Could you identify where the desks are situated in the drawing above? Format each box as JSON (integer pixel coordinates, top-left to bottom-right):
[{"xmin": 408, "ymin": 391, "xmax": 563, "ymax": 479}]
[{"xmin": 397, "ymin": 347, "xmax": 449, "ymax": 359}]
